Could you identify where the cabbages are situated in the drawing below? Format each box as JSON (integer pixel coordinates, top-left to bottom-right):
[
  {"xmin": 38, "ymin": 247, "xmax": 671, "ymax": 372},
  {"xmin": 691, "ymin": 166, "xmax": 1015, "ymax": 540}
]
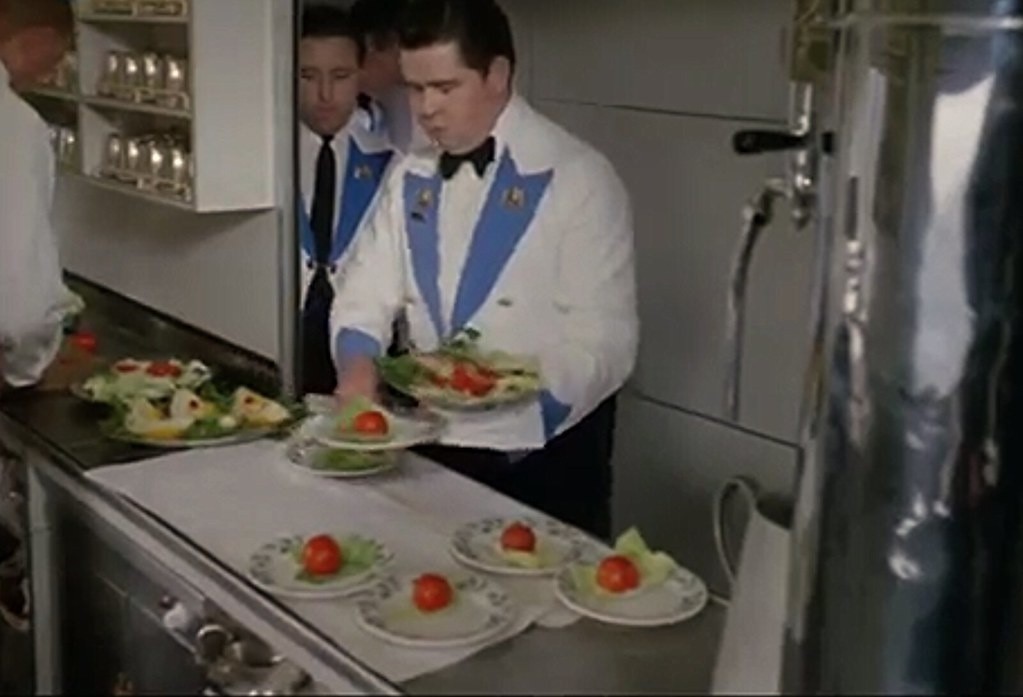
[{"xmin": 287, "ymin": 524, "xmax": 680, "ymax": 622}]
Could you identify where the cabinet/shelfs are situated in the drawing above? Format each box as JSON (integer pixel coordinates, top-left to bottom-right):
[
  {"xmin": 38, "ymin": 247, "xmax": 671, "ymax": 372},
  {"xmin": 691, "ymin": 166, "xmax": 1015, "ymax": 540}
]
[{"xmin": 21, "ymin": 0, "xmax": 275, "ymax": 213}]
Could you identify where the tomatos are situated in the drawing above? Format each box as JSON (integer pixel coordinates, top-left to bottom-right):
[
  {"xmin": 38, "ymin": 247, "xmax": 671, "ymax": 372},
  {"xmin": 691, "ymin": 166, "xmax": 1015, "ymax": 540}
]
[
  {"xmin": 354, "ymin": 409, "xmax": 387, "ymax": 434},
  {"xmin": 500, "ymin": 522, "xmax": 536, "ymax": 551},
  {"xmin": 596, "ymin": 556, "xmax": 638, "ymax": 592},
  {"xmin": 452, "ymin": 364, "xmax": 486, "ymax": 396},
  {"xmin": 412, "ymin": 573, "xmax": 450, "ymax": 611},
  {"xmin": 72, "ymin": 329, "xmax": 97, "ymax": 351},
  {"xmin": 303, "ymin": 534, "xmax": 340, "ymax": 573}
]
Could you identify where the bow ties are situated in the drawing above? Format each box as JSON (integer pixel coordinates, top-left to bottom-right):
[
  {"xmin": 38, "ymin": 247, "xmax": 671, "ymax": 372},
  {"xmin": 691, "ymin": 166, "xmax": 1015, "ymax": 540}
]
[{"xmin": 439, "ymin": 136, "xmax": 495, "ymax": 179}]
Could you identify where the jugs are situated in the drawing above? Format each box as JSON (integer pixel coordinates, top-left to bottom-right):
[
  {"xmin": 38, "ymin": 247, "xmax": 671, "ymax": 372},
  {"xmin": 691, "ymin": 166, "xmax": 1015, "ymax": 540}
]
[{"xmin": 707, "ymin": 475, "xmax": 794, "ymax": 697}]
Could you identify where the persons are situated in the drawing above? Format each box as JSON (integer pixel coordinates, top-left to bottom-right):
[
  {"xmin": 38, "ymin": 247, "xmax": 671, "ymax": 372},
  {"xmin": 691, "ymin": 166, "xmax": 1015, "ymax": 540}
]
[
  {"xmin": 299, "ymin": 0, "xmax": 417, "ymax": 413},
  {"xmin": 328, "ymin": 0, "xmax": 640, "ymax": 547},
  {"xmin": 0, "ymin": 0, "xmax": 85, "ymax": 388}
]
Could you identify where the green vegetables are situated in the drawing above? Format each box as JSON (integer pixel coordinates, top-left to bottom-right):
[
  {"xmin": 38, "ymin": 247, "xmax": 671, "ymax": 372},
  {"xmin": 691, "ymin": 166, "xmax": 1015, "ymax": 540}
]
[{"xmin": 81, "ymin": 339, "xmax": 538, "ymax": 470}]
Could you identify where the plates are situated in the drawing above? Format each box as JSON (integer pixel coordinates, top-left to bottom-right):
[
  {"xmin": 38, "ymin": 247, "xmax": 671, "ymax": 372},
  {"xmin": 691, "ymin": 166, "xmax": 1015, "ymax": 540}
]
[
  {"xmin": 449, "ymin": 519, "xmax": 586, "ymax": 576},
  {"xmin": 386, "ymin": 376, "xmax": 540, "ymax": 412},
  {"xmin": 551, "ymin": 553, "xmax": 708, "ymax": 626},
  {"xmin": 313, "ymin": 409, "xmax": 449, "ymax": 451},
  {"xmin": 245, "ymin": 532, "xmax": 397, "ymax": 600},
  {"xmin": 353, "ymin": 572, "xmax": 515, "ymax": 647},
  {"xmin": 68, "ymin": 368, "xmax": 268, "ymax": 448},
  {"xmin": 285, "ymin": 438, "xmax": 401, "ymax": 478}
]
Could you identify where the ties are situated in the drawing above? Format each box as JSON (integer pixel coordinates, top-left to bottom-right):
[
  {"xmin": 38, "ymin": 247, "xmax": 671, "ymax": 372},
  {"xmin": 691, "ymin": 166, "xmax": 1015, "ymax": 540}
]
[{"xmin": 309, "ymin": 135, "xmax": 336, "ymax": 266}]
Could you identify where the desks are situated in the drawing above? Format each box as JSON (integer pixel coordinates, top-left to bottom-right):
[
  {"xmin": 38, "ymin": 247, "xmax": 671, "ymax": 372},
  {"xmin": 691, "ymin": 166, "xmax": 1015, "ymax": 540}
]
[{"xmin": 0, "ymin": 304, "xmax": 731, "ymax": 697}]
